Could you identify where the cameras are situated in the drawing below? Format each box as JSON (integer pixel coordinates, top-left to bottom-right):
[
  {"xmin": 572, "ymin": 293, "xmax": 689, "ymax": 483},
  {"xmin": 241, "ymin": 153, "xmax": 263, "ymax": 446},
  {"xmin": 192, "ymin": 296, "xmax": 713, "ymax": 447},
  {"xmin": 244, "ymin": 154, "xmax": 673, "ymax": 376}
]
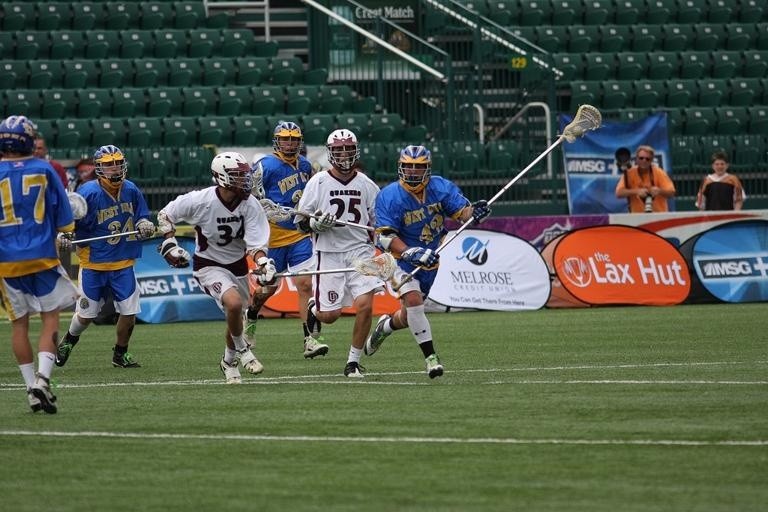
[{"xmin": 645, "ymin": 195, "xmax": 652, "ymax": 212}]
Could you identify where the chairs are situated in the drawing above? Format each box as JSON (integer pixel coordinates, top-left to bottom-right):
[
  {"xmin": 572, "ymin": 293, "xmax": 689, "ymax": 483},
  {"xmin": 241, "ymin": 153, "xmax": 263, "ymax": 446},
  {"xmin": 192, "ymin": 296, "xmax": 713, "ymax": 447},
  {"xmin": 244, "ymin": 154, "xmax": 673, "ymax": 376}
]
[
  {"xmin": 372, "ymin": 113, "xmax": 427, "ymax": 142},
  {"xmin": 584, "ymin": 1, "xmax": 617, "ymax": 26},
  {"xmin": 425, "ymin": 0, "xmax": 454, "ymax": 30},
  {"xmin": 386, "ymin": 142, "xmax": 406, "ymax": 174},
  {"xmin": 34, "ymin": 120, "xmax": 54, "ymax": 147},
  {"xmin": 51, "ymin": 30, "xmax": 86, "ymax": 59},
  {"xmin": 122, "ymin": 149, "xmax": 159, "ymax": 185},
  {"xmin": 38, "ymin": 0, "xmax": 72, "ymax": 30},
  {"xmin": 663, "ymin": 24, "xmax": 697, "ymax": 50},
  {"xmin": 143, "ymin": 147, "xmax": 196, "ymax": 186},
  {"xmin": 197, "ymin": 117, "xmax": 234, "ymax": 147},
  {"xmin": 274, "ymin": 57, "xmax": 328, "ymax": 84},
  {"xmin": 667, "ymin": 109, "xmax": 685, "ymax": 133},
  {"xmin": 586, "ymin": 51, "xmax": 616, "ymax": 81},
  {"xmin": 554, "ymin": 53, "xmax": 587, "ymax": 80},
  {"xmin": 221, "ymin": 29, "xmax": 280, "ymax": 56},
  {"xmin": 680, "ymin": 51, "xmax": 714, "ymax": 77},
  {"xmin": 318, "ymin": 86, "xmax": 378, "ymax": 114},
  {"xmin": 729, "ymin": 78, "xmax": 761, "ymax": 105},
  {"xmin": 335, "ymin": 114, "xmax": 372, "ymax": 144},
  {"xmin": 87, "ymin": 29, "xmax": 123, "ymax": 58},
  {"xmin": 141, "ymin": 0, "xmax": 175, "ymax": 29},
  {"xmin": 672, "ymin": 135, "xmax": 699, "ymax": 172},
  {"xmin": 454, "ymin": 143, "xmax": 508, "ymax": 179},
  {"xmin": 571, "ymin": 81, "xmax": 604, "ymax": 109},
  {"xmin": 426, "ymin": 140, "xmax": 476, "ymax": 180},
  {"xmin": 118, "ymin": 29, "xmax": 162, "ymax": 59},
  {"xmin": 616, "ymin": 0, "xmax": 648, "ymax": 24},
  {"xmin": 187, "ymin": 29, "xmax": 226, "ymax": 58},
  {"xmin": 487, "ymin": 0, "xmax": 519, "ymax": 25},
  {"xmin": 761, "ymin": 79, "xmax": 767, "ymax": 105},
  {"xmin": 537, "ymin": 25, "xmax": 569, "ymax": 53},
  {"xmin": 756, "ymin": 22, "xmax": 767, "ymax": 49},
  {"xmin": 73, "ymin": 2, "xmax": 107, "ymax": 28},
  {"xmin": 489, "ymin": 138, "xmax": 545, "ymax": 176},
  {"xmin": 567, "ymin": 24, "xmax": 608, "ymax": 51},
  {"xmin": 107, "ymin": 1, "xmax": 140, "ymax": 29},
  {"xmin": 1, "ymin": 30, "xmax": 14, "ymax": 60},
  {"xmin": 40, "ymin": 90, "xmax": 78, "ymax": 118},
  {"xmin": 601, "ymin": 81, "xmax": 635, "ymax": 109},
  {"xmin": 709, "ymin": 0, "xmax": 738, "ymax": 22},
  {"xmin": 97, "ymin": 60, "xmax": 138, "ymax": 87},
  {"xmin": 622, "ymin": 112, "xmax": 649, "ymax": 120},
  {"xmin": 163, "ymin": 117, "xmax": 199, "ymax": 147},
  {"xmin": 50, "ymin": 149, "xmax": 68, "ymax": 161},
  {"xmin": 220, "ymin": 86, "xmax": 253, "ymax": 115},
  {"xmin": 739, "ymin": 0, "xmax": 768, "ymax": 22},
  {"xmin": 56, "ymin": 119, "xmax": 92, "ymax": 147},
  {"xmin": 635, "ymin": 81, "xmax": 668, "ymax": 109},
  {"xmin": 235, "ymin": 116, "xmax": 269, "ymax": 146},
  {"xmin": 358, "ymin": 144, "xmax": 395, "ymax": 180},
  {"xmin": 6, "ymin": 88, "xmax": 44, "ymax": 118},
  {"xmin": 748, "ymin": 105, "xmax": 767, "ymax": 134},
  {"xmin": 668, "ymin": 78, "xmax": 699, "ymax": 108},
  {"xmin": 237, "ymin": 57, "xmax": 275, "ymax": 84},
  {"xmin": 714, "ymin": 107, "xmax": 747, "ymax": 134},
  {"xmin": 154, "ymin": 30, "xmax": 190, "ymax": 59},
  {"xmin": 728, "ymin": 21, "xmax": 759, "ymax": 50},
  {"xmin": 633, "ymin": 25, "xmax": 662, "ymax": 51},
  {"xmin": 175, "ymin": 1, "xmax": 231, "ymax": 29},
  {"xmin": 203, "ymin": 58, "xmax": 238, "ymax": 85},
  {"xmin": 475, "ymin": 27, "xmax": 499, "ymax": 62},
  {"xmin": 455, "ymin": 0, "xmax": 487, "ymax": 28},
  {"xmin": 745, "ymin": 51, "xmax": 768, "ymax": 78},
  {"xmin": 266, "ymin": 116, "xmax": 303, "ymax": 146},
  {"xmin": 3, "ymin": 2, "xmax": 36, "ymax": 28},
  {"xmin": 28, "ymin": 59, "xmax": 65, "ymax": 88},
  {"xmin": 616, "ymin": 51, "xmax": 648, "ymax": 80},
  {"xmin": 285, "ymin": 86, "xmax": 321, "ymax": 115},
  {"xmin": 148, "ymin": 87, "xmax": 185, "ymax": 116},
  {"xmin": 712, "ymin": 51, "xmax": 744, "ymax": 78},
  {"xmin": 70, "ymin": 149, "xmax": 90, "ymax": 161},
  {"xmin": 521, "ymin": 1, "xmax": 555, "ymax": 25},
  {"xmin": 90, "ymin": 118, "xmax": 127, "ymax": 147},
  {"xmin": 732, "ymin": 133, "xmax": 768, "ymax": 170},
  {"xmin": 250, "ymin": 86, "xmax": 288, "ymax": 115},
  {"xmin": 12, "ymin": 30, "xmax": 51, "ymax": 59},
  {"xmin": 694, "ymin": 23, "xmax": 729, "ymax": 50},
  {"xmin": 698, "ymin": 78, "xmax": 730, "ymax": 107},
  {"xmin": 129, "ymin": 117, "xmax": 165, "ymax": 148},
  {"xmin": 168, "ymin": 60, "xmax": 206, "ymax": 86},
  {"xmin": 133, "ymin": 60, "xmax": 170, "ymax": 87},
  {"xmin": 553, "ymin": 0, "xmax": 585, "ymax": 25},
  {"xmin": 75, "ymin": 88, "xmax": 114, "ymax": 118},
  {"xmin": 646, "ymin": 0, "xmax": 679, "ymax": 24},
  {"xmin": 62, "ymin": 60, "xmax": 101, "ymax": 88},
  {"xmin": 0, "ymin": 60, "xmax": 30, "ymax": 88},
  {"xmin": 649, "ymin": 50, "xmax": 680, "ymax": 78},
  {"xmin": 110, "ymin": 87, "xmax": 147, "ymax": 117},
  {"xmin": 677, "ymin": 0, "xmax": 710, "ymax": 24},
  {"xmin": 496, "ymin": 25, "xmax": 535, "ymax": 62},
  {"xmin": 686, "ymin": 108, "xmax": 716, "ymax": 133},
  {"xmin": 701, "ymin": 135, "xmax": 755, "ymax": 172},
  {"xmin": 185, "ymin": 87, "xmax": 219, "ymax": 116},
  {"xmin": 302, "ymin": 114, "xmax": 336, "ymax": 145},
  {"xmin": 180, "ymin": 147, "xmax": 218, "ymax": 186},
  {"xmin": 600, "ymin": 24, "xmax": 632, "ymax": 51}
]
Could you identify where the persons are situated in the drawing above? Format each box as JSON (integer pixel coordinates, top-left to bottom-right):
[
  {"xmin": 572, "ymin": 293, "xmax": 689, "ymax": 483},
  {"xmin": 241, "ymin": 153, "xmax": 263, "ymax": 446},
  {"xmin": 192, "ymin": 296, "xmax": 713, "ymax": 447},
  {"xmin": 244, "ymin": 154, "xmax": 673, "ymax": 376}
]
[
  {"xmin": 615, "ymin": 145, "xmax": 676, "ymax": 212},
  {"xmin": 1, "ymin": 116, "xmax": 78, "ymax": 415},
  {"xmin": 157, "ymin": 152, "xmax": 278, "ymax": 383},
  {"xmin": 67, "ymin": 158, "xmax": 97, "ymax": 192},
  {"xmin": 292, "ymin": 129, "xmax": 381, "ymax": 380},
  {"xmin": 363, "ymin": 143, "xmax": 491, "ymax": 381},
  {"xmin": 54, "ymin": 143, "xmax": 157, "ymax": 369},
  {"xmin": 694, "ymin": 152, "xmax": 746, "ymax": 210},
  {"xmin": 33, "ymin": 131, "xmax": 69, "ymax": 192},
  {"xmin": 243, "ymin": 120, "xmax": 331, "ymax": 361}
]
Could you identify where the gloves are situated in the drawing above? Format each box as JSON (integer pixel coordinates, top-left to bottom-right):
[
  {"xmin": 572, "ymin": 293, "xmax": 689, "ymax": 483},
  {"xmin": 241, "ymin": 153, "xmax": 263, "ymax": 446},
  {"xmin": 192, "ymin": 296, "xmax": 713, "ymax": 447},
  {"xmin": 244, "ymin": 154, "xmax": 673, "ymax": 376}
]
[
  {"xmin": 469, "ymin": 198, "xmax": 493, "ymax": 226},
  {"xmin": 299, "ymin": 209, "xmax": 339, "ymax": 234},
  {"xmin": 132, "ymin": 215, "xmax": 155, "ymax": 238},
  {"xmin": 157, "ymin": 234, "xmax": 193, "ymax": 273},
  {"xmin": 53, "ymin": 229, "xmax": 73, "ymax": 261},
  {"xmin": 250, "ymin": 255, "xmax": 279, "ymax": 288},
  {"xmin": 400, "ymin": 246, "xmax": 441, "ymax": 271}
]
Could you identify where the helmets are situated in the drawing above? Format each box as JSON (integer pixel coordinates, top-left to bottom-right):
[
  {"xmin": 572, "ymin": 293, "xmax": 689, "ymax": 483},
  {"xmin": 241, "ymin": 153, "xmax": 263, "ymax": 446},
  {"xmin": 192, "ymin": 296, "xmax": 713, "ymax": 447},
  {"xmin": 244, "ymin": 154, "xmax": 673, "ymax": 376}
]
[
  {"xmin": 396, "ymin": 145, "xmax": 433, "ymax": 196},
  {"xmin": 90, "ymin": 144, "xmax": 131, "ymax": 192},
  {"xmin": 208, "ymin": 150, "xmax": 256, "ymax": 202},
  {"xmin": 0, "ymin": 108, "xmax": 36, "ymax": 156},
  {"xmin": 325, "ymin": 127, "xmax": 362, "ymax": 176},
  {"xmin": 270, "ymin": 120, "xmax": 304, "ymax": 162}
]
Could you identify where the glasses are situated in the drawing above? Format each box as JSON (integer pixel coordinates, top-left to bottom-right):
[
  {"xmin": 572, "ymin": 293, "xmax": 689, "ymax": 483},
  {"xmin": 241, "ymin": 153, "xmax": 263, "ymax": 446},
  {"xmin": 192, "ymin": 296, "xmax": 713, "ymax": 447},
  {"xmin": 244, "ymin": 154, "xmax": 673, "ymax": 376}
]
[{"xmin": 635, "ymin": 155, "xmax": 652, "ymax": 162}]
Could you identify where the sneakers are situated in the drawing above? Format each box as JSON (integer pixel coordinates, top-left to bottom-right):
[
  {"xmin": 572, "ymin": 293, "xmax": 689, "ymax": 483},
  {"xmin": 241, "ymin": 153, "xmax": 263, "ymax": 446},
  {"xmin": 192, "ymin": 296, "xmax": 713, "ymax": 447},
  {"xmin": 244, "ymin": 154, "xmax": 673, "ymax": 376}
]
[
  {"xmin": 341, "ymin": 361, "xmax": 367, "ymax": 381},
  {"xmin": 423, "ymin": 351, "xmax": 446, "ymax": 378},
  {"xmin": 22, "ymin": 384, "xmax": 58, "ymax": 412},
  {"xmin": 301, "ymin": 334, "xmax": 331, "ymax": 361},
  {"xmin": 53, "ymin": 329, "xmax": 81, "ymax": 368},
  {"xmin": 234, "ymin": 345, "xmax": 266, "ymax": 378},
  {"xmin": 219, "ymin": 352, "xmax": 245, "ymax": 389},
  {"xmin": 240, "ymin": 306, "xmax": 260, "ymax": 351},
  {"xmin": 362, "ymin": 312, "xmax": 395, "ymax": 357},
  {"xmin": 301, "ymin": 296, "xmax": 320, "ymax": 337},
  {"xmin": 28, "ymin": 372, "xmax": 58, "ymax": 417},
  {"xmin": 110, "ymin": 345, "xmax": 141, "ymax": 370}
]
